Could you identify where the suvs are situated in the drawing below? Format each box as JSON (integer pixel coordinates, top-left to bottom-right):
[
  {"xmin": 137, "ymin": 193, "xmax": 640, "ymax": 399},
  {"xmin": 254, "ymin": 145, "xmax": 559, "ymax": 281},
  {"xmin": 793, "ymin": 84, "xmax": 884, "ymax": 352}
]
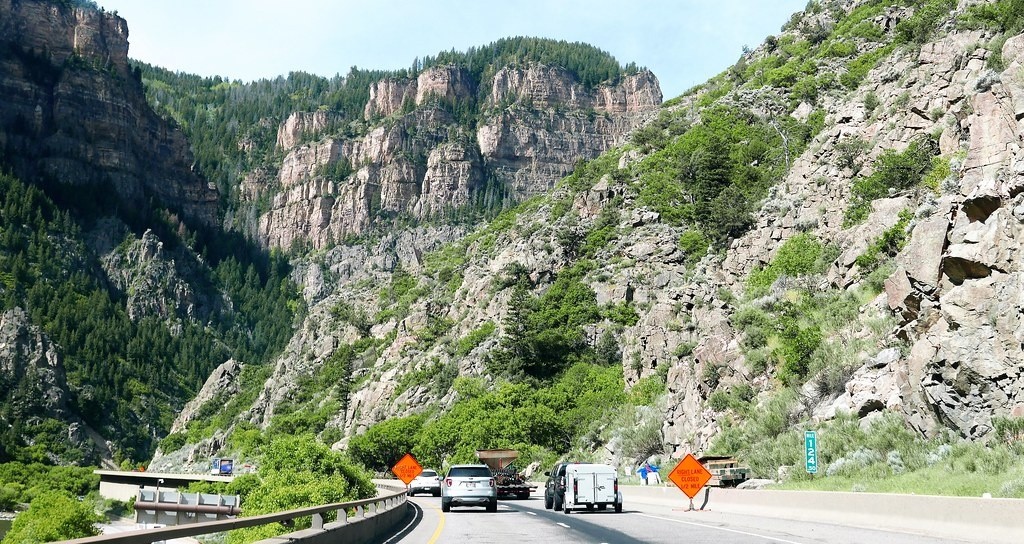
[
  {"xmin": 544, "ymin": 461, "xmax": 595, "ymax": 510},
  {"xmin": 438, "ymin": 463, "xmax": 499, "ymax": 513},
  {"xmin": 560, "ymin": 463, "xmax": 623, "ymax": 514}
]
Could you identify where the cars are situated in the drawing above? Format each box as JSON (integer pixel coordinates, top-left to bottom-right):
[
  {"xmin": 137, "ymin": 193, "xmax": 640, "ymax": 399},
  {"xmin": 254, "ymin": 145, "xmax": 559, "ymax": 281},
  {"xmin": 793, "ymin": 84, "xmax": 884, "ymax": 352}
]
[{"xmin": 406, "ymin": 469, "xmax": 442, "ymax": 497}]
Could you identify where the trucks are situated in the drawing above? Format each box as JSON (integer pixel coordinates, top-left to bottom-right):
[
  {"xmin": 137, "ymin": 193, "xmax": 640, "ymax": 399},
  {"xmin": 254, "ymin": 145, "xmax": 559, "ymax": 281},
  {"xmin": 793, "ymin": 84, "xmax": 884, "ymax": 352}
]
[
  {"xmin": 475, "ymin": 448, "xmax": 539, "ymax": 500},
  {"xmin": 208, "ymin": 458, "xmax": 234, "ymax": 477}
]
